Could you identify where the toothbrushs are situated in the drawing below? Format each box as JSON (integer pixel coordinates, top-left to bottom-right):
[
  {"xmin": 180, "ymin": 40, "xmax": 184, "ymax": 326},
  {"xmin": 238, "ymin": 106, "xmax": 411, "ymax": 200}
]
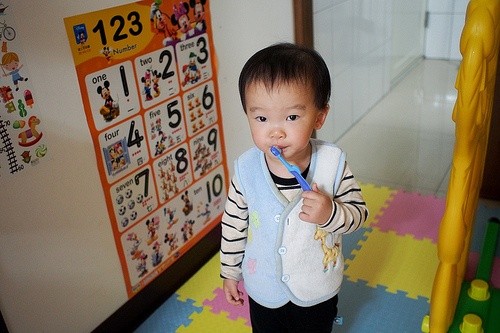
[{"xmin": 270, "ymin": 145, "xmax": 313, "ymax": 192}]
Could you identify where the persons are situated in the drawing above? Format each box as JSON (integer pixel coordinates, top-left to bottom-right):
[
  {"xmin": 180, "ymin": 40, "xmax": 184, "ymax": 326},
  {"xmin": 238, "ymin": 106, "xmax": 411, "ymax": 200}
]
[{"xmin": 220, "ymin": 42, "xmax": 368, "ymax": 333}]
